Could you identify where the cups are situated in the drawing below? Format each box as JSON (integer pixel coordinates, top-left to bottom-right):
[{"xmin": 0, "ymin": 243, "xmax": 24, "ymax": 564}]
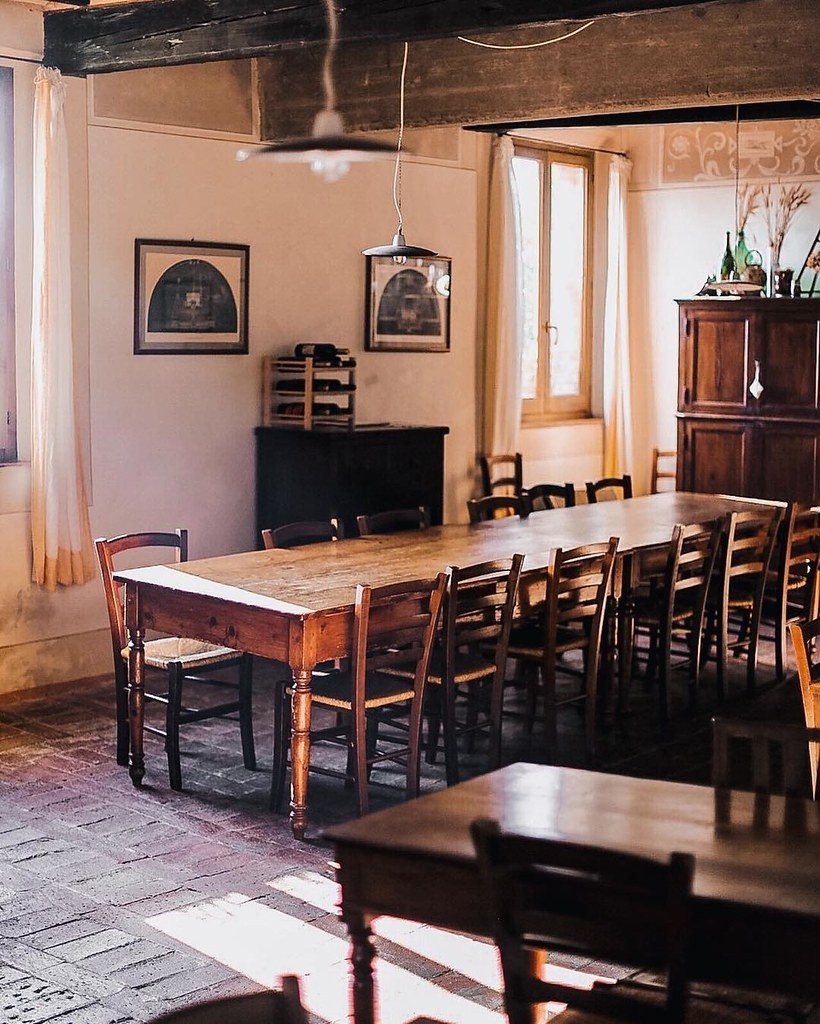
[{"xmin": 774, "ymin": 271, "xmax": 794, "ymax": 299}]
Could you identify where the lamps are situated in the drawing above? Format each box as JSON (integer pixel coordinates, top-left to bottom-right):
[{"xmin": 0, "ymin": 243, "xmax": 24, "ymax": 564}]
[
  {"xmin": 706, "ymin": 103, "xmax": 763, "ymax": 294},
  {"xmin": 237, "ymin": 0, "xmax": 408, "ymax": 182},
  {"xmin": 359, "ymin": 42, "xmax": 438, "ymax": 267}
]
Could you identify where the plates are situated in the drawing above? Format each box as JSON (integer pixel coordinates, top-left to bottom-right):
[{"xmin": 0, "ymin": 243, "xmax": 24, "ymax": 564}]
[{"xmin": 713, "ymin": 281, "xmax": 763, "ymax": 295}]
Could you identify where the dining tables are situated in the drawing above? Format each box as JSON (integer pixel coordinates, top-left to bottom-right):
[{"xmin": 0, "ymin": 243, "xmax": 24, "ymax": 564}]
[
  {"xmin": 316, "ymin": 762, "xmax": 820, "ymax": 1024},
  {"xmin": 111, "ymin": 490, "xmax": 814, "ymax": 788}
]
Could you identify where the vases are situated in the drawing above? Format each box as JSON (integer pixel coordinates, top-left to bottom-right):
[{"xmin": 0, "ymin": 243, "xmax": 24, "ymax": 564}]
[
  {"xmin": 769, "ymin": 263, "xmax": 780, "ymax": 298},
  {"xmin": 734, "ymin": 231, "xmax": 766, "ymax": 284},
  {"xmin": 720, "ymin": 231, "xmax": 736, "ymax": 277}
]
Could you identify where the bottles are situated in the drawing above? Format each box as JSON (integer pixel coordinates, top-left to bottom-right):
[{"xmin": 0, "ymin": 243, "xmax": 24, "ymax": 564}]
[
  {"xmin": 295, "ymin": 343, "xmax": 349, "ymax": 361},
  {"xmin": 276, "ymin": 403, "xmax": 353, "ymax": 420},
  {"xmin": 277, "ymin": 379, "xmax": 355, "ymax": 397},
  {"xmin": 276, "ymin": 357, "xmax": 356, "ymax": 373},
  {"xmin": 720, "ymin": 232, "xmax": 737, "ymax": 296}
]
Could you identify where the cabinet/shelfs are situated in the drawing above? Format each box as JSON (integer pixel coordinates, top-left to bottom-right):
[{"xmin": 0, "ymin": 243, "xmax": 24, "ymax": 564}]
[
  {"xmin": 262, "ymin": 355, "xmax": 357, "ymax": 432},
  {"xmin": 255, "ymin": 422, "xmax": 450, "ymax": 669},
  {"xmin": 673, "ymin": 296, "xmax": 819, "ymax": 597}
]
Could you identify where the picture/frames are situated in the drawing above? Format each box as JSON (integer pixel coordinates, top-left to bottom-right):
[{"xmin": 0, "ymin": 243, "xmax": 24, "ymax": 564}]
[
  {"xmin": 364, "ymin": 253, "xmax": 452, "ymax": 352},
  {"xmin": 134, "ymin": 238, "xmax": 250, "ymax": 355}
]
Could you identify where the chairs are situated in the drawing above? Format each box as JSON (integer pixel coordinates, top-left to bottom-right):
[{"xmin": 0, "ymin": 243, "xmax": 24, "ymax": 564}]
[
  {"xmin": 467, "ymin": 452, "xmax": 633, "ymax": 525},
  {"xmin": 356, "ymin": 506, "xmax": 498, "ymax": 762},
  {"xmin": 355, "ymin": 553, "xmax": 524, "ymax": 787},
  {"xmin": 468, "ymin": 537, "xmax": 621, "ymax": 763},
  {"xmin": 470, "ymin": 816, "xmax": 757, "ymax": 1024},
  {"xmin": 789, "ymin": 618, "xmax": 820, "ymax": 803},
  {"xmin": 644, "ymin": 507, "xmax": 785, "ymax": 696},
  {"xmin": 94, "ymin": 528, "xmax": 258, "ymax": 787},
  {"xmin": 649, "ymin": 448, "xmax": 677, "ymax": 496},
  {"xmin": 624, "ymin": 716, "xmax": 820, "ymax": 1024},
  {"xmin": 261, "ymin": 517, "xmax": 422, "ymax": 743},
  {"xmin": 614, "ymin": 516, "xmax": 727, "ymax": 728},
  {"xmin": 143, "ymin": 976, "xmax": 307, "ymax": 1024},
  {"xmin": 270, "ymin": 573, "xmax": 449, "ymax": 818},
  {"xmin": 733, "ymin": 502, "xmax": 819, "ymax": 677}
]
